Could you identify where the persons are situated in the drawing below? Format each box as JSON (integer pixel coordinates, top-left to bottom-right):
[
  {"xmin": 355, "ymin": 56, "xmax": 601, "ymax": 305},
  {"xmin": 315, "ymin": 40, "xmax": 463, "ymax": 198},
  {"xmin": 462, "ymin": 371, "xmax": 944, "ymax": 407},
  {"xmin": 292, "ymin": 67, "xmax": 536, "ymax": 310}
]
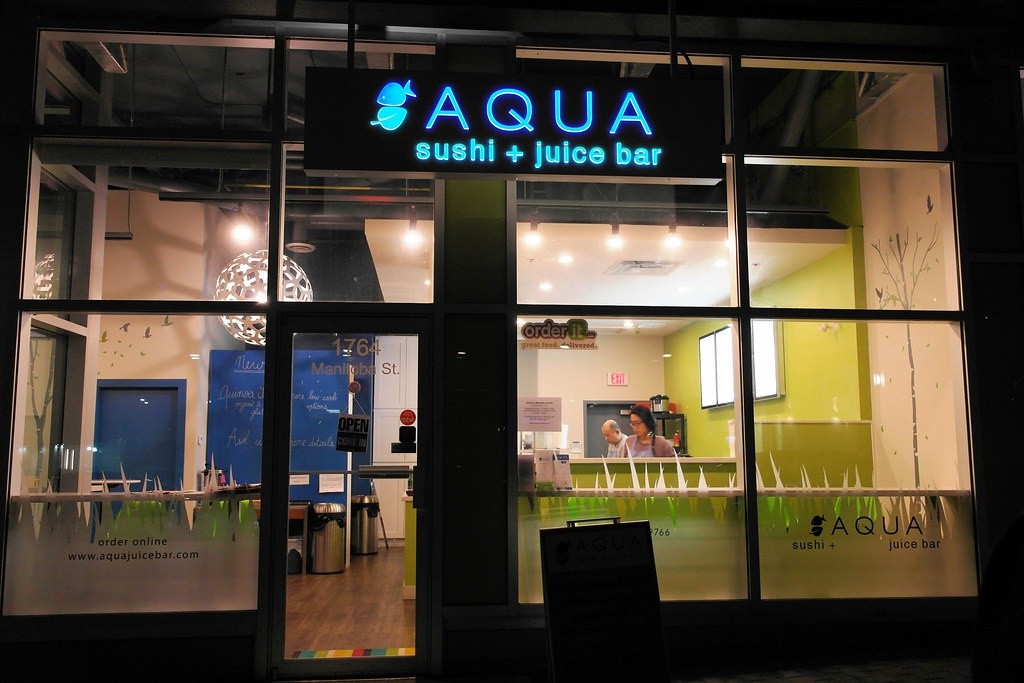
[
  {"xmin": 602, "ymin": 419, "xmax": 628, "ymax": 457},
  {"xmin": 623, "ymin": 405, "xmax": 674, "ymax": 457}
]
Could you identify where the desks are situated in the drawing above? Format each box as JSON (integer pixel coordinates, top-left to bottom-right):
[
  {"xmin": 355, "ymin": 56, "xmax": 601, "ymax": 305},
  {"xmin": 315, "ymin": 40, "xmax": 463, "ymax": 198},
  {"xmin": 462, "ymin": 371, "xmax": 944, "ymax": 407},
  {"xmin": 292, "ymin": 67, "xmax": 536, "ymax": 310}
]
[
  {"xmin": 517, "ymin": 488, "xmax": 970, "ymax": 599},
  {"xmin": 12, "ymin": 487, "xmax": 261, "ymax": 542}
]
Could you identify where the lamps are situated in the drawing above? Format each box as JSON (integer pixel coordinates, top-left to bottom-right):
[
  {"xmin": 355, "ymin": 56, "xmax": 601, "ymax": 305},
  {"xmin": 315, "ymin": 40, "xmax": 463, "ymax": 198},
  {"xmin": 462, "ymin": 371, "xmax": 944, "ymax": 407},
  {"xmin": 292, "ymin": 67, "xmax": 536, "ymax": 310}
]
[
  {"xmin": 214, "ymin": 249, "xmax": 314, "ymax": 346},
  {"xmin": 105, "ymin": 190, "xmax": 133, "ymax": 240}
]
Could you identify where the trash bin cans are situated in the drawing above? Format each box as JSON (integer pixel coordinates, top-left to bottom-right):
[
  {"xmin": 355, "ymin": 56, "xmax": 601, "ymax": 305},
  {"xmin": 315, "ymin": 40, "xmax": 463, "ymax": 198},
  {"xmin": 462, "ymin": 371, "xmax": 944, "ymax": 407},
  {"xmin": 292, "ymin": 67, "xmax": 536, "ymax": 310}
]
[
  {"xmin": 313, "ymin": 502, "xmax": 345, "ymax": 574},
  {"xmin": 351, "ymin": 495, "xmax": 380, "ymax": 554},
  {"xmin": 288, "ymin": 537, "xmax": 302, "ymax": 574}
]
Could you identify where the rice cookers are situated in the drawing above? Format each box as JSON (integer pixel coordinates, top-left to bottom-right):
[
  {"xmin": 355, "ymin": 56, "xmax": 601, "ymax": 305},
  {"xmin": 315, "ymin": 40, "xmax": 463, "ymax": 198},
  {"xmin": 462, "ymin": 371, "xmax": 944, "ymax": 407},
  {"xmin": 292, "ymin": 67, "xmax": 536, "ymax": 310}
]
[{"xmin": 649, "ymin": 394, "xmax": 669, "ymax": 414}]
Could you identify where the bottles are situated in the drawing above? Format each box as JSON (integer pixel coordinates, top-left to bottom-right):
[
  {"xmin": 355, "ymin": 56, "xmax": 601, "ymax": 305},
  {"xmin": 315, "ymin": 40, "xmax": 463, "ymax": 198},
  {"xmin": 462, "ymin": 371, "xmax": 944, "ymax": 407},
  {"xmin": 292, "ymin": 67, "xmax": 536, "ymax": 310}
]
[{"xmin": 674, "ymin": 433, "xmax": 679, "ymax": 447}]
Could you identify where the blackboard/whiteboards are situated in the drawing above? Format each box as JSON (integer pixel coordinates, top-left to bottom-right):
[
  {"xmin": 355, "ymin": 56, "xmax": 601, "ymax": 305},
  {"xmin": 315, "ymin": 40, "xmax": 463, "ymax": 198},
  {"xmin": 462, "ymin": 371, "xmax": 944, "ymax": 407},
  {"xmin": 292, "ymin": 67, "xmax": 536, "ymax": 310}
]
[{"xmin": 538, "ymin": 517, "xmax": 670, "ymax": 682}]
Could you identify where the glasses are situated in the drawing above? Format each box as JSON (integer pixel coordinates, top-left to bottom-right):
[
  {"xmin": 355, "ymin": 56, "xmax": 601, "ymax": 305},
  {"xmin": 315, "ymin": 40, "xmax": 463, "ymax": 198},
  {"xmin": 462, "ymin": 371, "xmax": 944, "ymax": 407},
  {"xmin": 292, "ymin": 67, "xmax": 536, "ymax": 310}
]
[{"xmin": 630, "ymin": 421, "xmax": 643, "ymax": 427}]
